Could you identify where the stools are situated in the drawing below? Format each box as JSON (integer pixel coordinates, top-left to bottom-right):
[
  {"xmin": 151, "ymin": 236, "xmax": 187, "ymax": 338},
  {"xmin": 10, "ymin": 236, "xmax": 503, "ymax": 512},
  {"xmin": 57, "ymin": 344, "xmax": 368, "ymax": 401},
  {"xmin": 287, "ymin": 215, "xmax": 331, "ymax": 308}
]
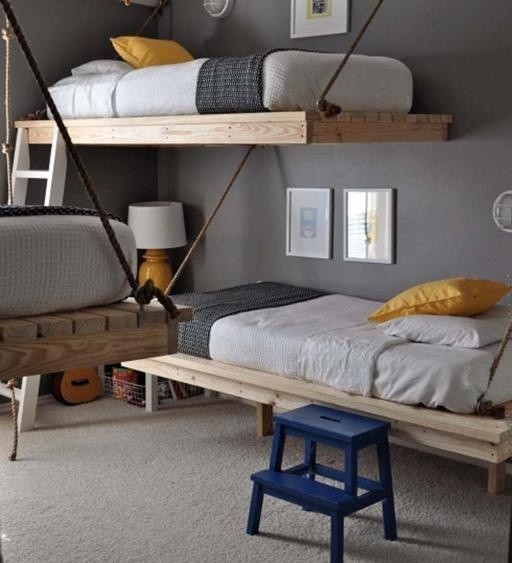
[{"xmin": 241, "ymin": 400, "xmax": 400, "ymax": 562}]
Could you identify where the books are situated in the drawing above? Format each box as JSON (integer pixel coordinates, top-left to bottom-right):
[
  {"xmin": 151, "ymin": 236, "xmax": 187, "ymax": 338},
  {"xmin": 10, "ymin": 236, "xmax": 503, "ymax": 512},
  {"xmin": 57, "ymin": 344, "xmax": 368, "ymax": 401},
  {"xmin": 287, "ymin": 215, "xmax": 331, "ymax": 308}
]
[{"xmin": 110, "ymin": 367, "xmax": 190, "ymax": 408}]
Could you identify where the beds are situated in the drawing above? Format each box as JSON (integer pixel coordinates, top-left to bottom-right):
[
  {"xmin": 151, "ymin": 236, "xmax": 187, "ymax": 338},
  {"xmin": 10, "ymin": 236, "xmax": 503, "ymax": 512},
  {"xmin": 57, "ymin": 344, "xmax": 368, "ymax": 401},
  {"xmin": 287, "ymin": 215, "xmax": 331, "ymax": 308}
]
[
  {"xmin": 121, "ymin": 281, "xmax": 511, "ymax": 499},
  {"xmin": 16, "ymin": 47, "xmax": 452, "ymax": 144},
  {"xmin": 2, "ymin": 203, "xmax": 192, "ymax": 379}
]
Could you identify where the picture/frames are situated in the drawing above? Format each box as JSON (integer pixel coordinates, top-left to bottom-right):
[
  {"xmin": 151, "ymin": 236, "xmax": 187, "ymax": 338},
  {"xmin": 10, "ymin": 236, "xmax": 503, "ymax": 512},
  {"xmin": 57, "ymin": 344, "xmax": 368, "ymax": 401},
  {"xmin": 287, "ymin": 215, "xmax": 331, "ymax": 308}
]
[
  {"xmin": 289, "ymin": 0, "xmax": 351, "ymax": 38},
  {"xmin": 342, "ymin": 187, "xmax": 396, "ymax": 264},
  {"xmin": 285, "ymin": 187, "xmax": 334, "ymax": 259}
]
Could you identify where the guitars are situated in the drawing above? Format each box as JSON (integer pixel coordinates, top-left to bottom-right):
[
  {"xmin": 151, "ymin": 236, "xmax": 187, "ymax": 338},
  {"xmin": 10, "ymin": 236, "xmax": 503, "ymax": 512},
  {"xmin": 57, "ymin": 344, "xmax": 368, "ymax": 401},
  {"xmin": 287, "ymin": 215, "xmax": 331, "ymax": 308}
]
[{"xmin": 52, "ymin": 365, "xmax": 103, "ymax": 404}]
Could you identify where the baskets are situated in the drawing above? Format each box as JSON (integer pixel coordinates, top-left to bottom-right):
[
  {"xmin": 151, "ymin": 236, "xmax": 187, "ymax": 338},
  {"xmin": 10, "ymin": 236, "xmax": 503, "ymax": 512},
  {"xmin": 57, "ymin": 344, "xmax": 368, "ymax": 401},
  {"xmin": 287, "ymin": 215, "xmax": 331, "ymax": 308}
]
[{"xmin": 100, "ymin": 373, "xmax": 204, "ymax": 408}]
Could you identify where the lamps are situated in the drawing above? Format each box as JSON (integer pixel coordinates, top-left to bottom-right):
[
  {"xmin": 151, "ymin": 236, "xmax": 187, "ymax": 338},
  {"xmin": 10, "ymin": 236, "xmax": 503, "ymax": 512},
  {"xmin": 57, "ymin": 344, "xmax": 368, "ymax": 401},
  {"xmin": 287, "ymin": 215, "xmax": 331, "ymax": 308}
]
[
  {"xmin": 202, "ymin": 0, "xmax": 235, "ymax": 21},
  {"xmin": 127, "ymin": 200, "xmax": 189, "ymax": 296},
  {"xmin": 490, "ymin": 188, "xmax": 511, "ymax": 234}
]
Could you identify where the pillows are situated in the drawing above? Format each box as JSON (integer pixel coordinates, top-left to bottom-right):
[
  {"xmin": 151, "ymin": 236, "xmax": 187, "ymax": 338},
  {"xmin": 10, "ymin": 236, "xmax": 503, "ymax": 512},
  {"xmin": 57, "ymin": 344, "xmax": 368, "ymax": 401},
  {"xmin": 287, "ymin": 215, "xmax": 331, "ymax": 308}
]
[
  {"xmin": 71, "ymin": 59, "xmax": 133, "ymax": 74},
  {"xmin": 374, "ymin": 304, "xmax": 511, "ymax": 348},
  {"xmin": 108, "ymin": 35, "xmax": 196, "ymax": 68},
  {"xmin": 366, "ymin": 276, "xmax": 511, "ymax": 323}
]
